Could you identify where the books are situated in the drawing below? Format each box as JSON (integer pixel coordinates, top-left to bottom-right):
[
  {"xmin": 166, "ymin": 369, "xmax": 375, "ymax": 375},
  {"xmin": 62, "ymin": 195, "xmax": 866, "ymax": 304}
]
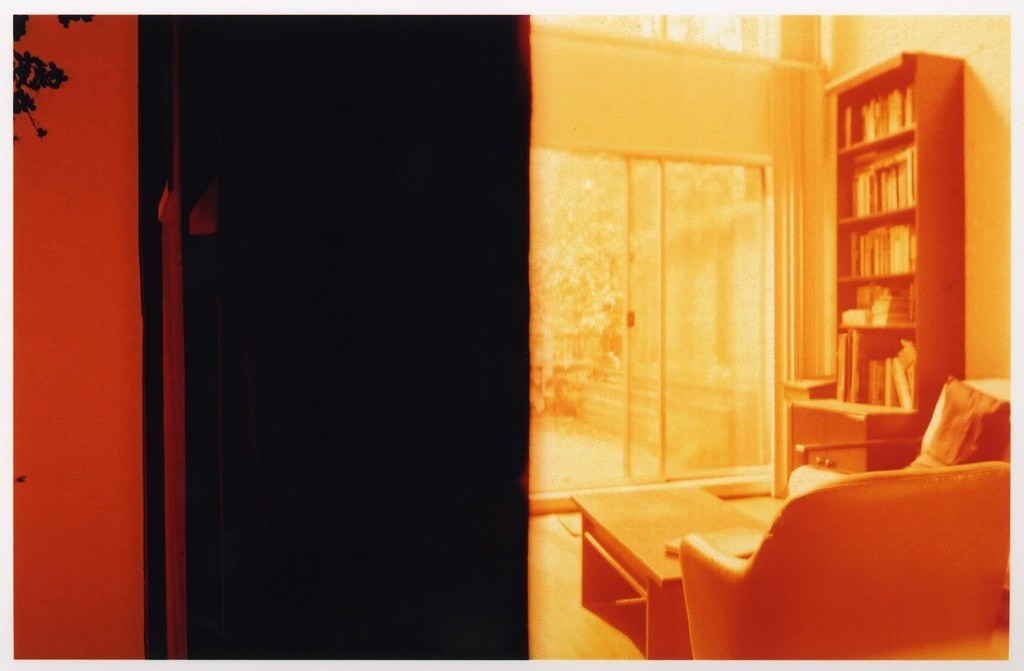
[
  {"xmin": 842, "ymin": 282, "xmax": 914, "ymax": 324},
  {"xmin": 850, "ymin": 223, "xmax": 917, "ymax": 274},
  {"xmin": 846, "ymin": 84, "xmax": 915, "ymax": 143},
  {"xmin": 837, "ymin": 331, "xmax": 914, "ymax": 409},
  {"xmin": 851, "ymin": 144, "xmax": 920, "ymax": 217}
]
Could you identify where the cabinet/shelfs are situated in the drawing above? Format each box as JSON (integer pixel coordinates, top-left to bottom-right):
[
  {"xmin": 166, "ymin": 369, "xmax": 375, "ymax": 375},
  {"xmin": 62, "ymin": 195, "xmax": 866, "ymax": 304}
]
[{"xmin": 787, "ymin": 53, "xmax": 965, "ymax": 470}]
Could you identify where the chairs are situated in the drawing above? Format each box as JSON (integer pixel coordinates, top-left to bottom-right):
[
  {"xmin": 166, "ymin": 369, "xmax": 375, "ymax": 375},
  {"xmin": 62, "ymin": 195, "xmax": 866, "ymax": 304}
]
[{"xmin": 680, "ymin": 460, "xmax": 1011, "ymax": 660}]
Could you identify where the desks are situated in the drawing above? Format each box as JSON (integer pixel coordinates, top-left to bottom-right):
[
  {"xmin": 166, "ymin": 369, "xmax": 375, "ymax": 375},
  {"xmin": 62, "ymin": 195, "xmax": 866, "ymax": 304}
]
[{"xmin": 571, "ymin": 486, "xmax": 769, "ymax": 661}]
